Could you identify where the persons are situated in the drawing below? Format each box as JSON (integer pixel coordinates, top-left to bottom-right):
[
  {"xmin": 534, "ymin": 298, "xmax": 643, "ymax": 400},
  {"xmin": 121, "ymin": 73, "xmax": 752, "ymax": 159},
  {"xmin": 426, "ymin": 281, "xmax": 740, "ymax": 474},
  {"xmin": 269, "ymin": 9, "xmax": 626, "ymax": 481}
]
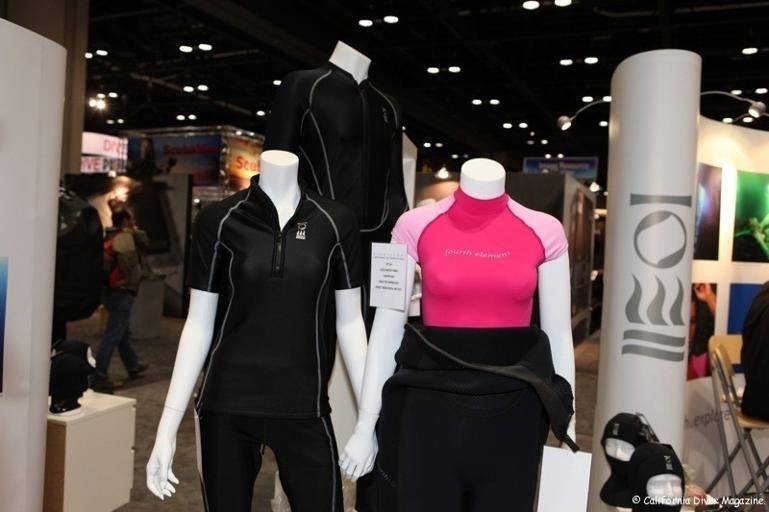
[
  {"xmin": 264, "ymin": 41, "xmax": 411, "ymax": 346},
  {"xmin": 145, "ymin": 150, "xmax": 368, "ymax": 511},
  {"xmin": 340, "ymin": 158, "xmax": 577, "ymax": 511},
  {"xmin": 687, "ymin": 282, "xmax": 716, "ymax": 379},
  {"xmin": 87, "ymin": 203, "xmax": 150, "ymax": 387},
  {"xmin": 739, "ymin": 280, "xmax": 768, "ymax": 425}
]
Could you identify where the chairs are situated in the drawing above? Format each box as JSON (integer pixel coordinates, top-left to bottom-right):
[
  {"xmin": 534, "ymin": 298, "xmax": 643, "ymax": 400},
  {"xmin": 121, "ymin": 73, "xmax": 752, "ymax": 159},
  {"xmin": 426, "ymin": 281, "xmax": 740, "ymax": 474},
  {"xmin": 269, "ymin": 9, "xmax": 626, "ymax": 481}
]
[
  {"xmin": 713, "ymin": 344, "xmax": 767, "ymax": 510},
  {"xmin": 702, "ymin": 329, "xmax": 767, "ymax": 501}
]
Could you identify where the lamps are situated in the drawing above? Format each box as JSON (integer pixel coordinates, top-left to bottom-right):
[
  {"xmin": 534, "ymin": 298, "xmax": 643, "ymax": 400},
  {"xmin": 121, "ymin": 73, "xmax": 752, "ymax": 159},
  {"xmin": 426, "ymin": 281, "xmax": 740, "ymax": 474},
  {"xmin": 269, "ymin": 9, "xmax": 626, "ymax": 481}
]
[
  {"xmin": 701, "ymin": 90, "xmax": 768, "ymax": 118},
  {"xmin": 558, "ymin": 96, "xmax": 617, "ymax": 135}
]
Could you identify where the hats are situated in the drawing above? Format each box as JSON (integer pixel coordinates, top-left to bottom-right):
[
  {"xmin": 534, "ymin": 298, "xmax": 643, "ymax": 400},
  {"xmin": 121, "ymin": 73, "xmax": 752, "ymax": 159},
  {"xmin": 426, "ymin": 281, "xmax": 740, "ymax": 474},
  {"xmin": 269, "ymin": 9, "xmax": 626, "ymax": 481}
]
[
  {"xmin": 50, "ymin": 339, "xmax": 98, "ymax": 398},
  {"xmin": 599, "ymin": 412, "xmax": 685, "ymax": 512}
]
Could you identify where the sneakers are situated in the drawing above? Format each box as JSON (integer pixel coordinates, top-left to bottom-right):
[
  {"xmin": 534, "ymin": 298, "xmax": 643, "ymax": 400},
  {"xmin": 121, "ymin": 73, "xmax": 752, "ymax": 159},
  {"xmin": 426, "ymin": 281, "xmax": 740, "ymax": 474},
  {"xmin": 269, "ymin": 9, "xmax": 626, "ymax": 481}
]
[
  {"xmin": 127, "ymin": 362, "xmax": 149, "ymax": 379},
  {"xmin": 91, "ymin": 380, "xmax": 113, "ymax": 393}
]
